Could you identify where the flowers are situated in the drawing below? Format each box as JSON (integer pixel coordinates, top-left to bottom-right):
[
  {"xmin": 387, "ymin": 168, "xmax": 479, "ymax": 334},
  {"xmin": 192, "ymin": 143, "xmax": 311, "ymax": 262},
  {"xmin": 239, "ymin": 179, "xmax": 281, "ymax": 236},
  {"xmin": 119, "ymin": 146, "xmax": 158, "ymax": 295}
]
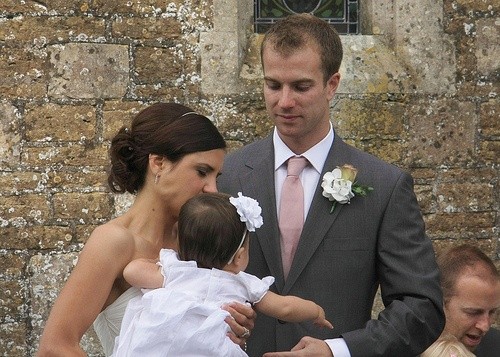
[
  {"xmin": 321, "ymin": 163, "xmax": 373, "ymax": 215},
  {"xmin": 230, "ymin": 190, "xmax": 263, "ymax": 232}
]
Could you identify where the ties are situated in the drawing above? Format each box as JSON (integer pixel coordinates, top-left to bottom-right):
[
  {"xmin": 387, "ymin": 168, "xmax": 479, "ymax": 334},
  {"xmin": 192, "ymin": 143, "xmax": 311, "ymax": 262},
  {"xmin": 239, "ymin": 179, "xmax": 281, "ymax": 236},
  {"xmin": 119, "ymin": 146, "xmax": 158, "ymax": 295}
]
[{"xmin": 278, "ymin": 156, "xmax": 308, "ymax": 279}]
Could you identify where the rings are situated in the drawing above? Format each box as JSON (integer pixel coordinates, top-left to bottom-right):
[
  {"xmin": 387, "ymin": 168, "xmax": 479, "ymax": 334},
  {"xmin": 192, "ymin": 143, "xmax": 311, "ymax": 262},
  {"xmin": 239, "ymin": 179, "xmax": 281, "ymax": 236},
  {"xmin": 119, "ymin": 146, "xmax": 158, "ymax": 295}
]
[
  {"xmin": 242, "ymin": 341, "xmax": 248, "ymax": 351},
  {"xmin": 239, "ymin": 327, "xmax": 250, "ymax": 337}
]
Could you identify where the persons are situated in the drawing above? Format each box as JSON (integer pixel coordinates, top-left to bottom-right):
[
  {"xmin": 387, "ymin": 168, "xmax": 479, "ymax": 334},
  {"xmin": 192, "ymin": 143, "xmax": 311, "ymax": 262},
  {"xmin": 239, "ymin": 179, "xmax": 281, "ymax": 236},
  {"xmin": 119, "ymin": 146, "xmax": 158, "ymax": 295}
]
[
  {"xmin": 216, "ymin": 13, "xmax": 445, "ymax": 357},
  {"xmin": 113, "ymin": 192, "xmax": 334, "ymax": 357},
  {"xmin": 36, "ymin": 102, "xmax": 258, "ymax": 357},
  {"xmin": 419, "ymin": 337, "xmax": 476, "ymax": 357},
  {"xmin": 435, "ymin": 244, "xmax": 500, "ymax": 351}
]
